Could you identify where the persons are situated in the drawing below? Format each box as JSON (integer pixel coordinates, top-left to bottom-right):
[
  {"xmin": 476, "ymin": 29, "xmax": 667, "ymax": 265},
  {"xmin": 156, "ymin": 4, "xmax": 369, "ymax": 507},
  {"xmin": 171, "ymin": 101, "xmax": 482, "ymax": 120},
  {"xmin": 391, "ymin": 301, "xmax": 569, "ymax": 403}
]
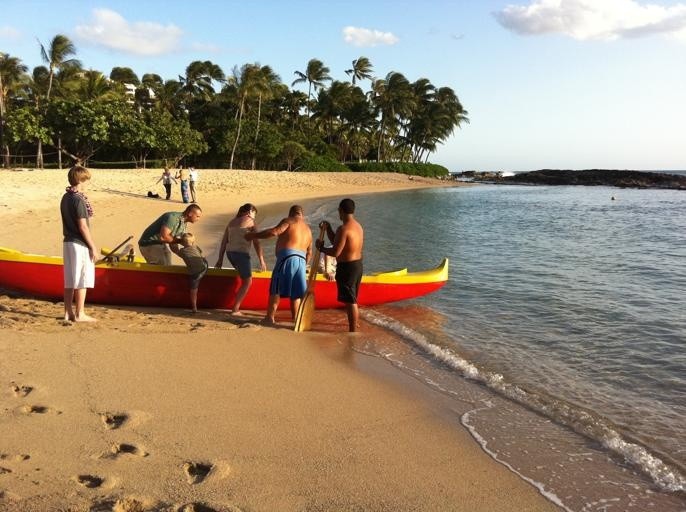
[
  {"xmin": 156, "ymin": 166, "xmax": 198, "ymax": 203},
  {"xmin": 138, "ymin": 198, "xmax": 364, "ymax": 332},
  {"xmin": 60, "ymin": 166, "xmax": 99, "ymax": 323}
]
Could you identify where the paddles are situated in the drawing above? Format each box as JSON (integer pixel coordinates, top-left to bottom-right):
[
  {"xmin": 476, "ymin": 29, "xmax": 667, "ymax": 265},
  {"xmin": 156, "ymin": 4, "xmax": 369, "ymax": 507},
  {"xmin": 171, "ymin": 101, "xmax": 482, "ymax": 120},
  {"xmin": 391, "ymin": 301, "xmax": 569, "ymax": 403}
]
[{"xmin": 295, "ymin": 222, "xmax": 326, "ymax": 332}]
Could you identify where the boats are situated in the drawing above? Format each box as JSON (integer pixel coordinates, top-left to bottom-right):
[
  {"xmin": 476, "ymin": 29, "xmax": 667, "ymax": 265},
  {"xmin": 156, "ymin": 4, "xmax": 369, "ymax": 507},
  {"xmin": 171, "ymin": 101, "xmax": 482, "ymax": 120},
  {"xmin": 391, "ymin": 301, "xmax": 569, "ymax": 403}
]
[{"xmin": 0, "ymin": 245, "xmax": 449, "ymax": 308}]
